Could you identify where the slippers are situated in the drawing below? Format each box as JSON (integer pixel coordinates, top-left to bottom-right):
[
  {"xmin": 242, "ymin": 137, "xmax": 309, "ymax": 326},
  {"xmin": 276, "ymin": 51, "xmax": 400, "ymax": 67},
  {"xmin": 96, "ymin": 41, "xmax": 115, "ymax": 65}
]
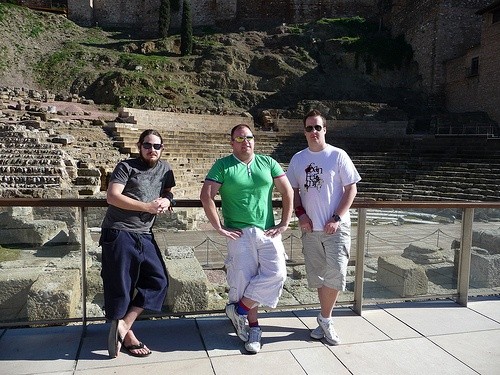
[
  {"xmin": 122, "ymin": 341, "xmax": 152, "ymax": 357},
  {"xmin": 107, "ymin": 318, "xmax": 123, "ymax": 358}
]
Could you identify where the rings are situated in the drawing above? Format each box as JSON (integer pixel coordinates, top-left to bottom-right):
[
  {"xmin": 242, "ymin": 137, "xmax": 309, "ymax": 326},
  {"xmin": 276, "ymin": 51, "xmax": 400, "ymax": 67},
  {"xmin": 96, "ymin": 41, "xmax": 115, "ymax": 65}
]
[{"xmin": 304, "ymin": 227, "xmax": 305, "ymax": 230}]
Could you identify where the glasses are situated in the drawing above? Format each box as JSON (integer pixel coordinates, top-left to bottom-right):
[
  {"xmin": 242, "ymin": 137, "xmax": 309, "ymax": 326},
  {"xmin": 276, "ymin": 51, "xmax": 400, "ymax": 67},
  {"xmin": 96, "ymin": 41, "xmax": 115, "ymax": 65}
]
[
  {"xmin": 140, "ymin": 143, "xmax": 164, "ymax": 150},
  {"xmin": 233, "ymin": 136, "xmax": 254, "ymax": 143},
  {"xmin": 305, "ymin": 125, "xmax": 325, "ymax": 132}
]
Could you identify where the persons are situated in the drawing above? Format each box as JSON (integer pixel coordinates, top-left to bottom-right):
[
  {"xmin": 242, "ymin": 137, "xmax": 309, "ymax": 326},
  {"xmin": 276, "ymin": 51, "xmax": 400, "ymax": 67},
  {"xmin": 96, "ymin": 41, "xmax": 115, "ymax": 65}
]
[
  {"xmin": 100, "ymin": 130, "xmax": 176, "ymax": 358},
  {"xmin": 287, "ymin": 109, "xmax": 362, "ymax": 345},
  {"xmin": 200, "ymin": 124, "xmax": 294, "ymax": 354}
]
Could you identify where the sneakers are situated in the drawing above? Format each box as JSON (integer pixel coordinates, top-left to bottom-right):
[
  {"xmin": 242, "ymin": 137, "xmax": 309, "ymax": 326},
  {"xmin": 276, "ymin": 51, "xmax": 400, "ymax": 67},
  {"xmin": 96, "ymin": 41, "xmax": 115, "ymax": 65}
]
[
  {"xmin": 310, "ymin": 324, "xmax": 325, "ymax": 338},
  {"xmin": 225, "ymin": 303, "xmax": 249, "ymax": 342},
  {"xmin": 244, "ymin": 326, "xmax": 262, "ymax": 353},
  {"xmin": 317, "ymin": 312, "xmax": 341, "ymax": 344}
]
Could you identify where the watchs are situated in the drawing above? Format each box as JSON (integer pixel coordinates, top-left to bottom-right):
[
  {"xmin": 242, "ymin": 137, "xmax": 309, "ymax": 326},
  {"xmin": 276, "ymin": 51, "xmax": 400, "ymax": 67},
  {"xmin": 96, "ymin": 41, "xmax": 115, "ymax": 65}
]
[{"xmin": 332, "ymin": 214, "xmax": 341, "ymax": 222}]
